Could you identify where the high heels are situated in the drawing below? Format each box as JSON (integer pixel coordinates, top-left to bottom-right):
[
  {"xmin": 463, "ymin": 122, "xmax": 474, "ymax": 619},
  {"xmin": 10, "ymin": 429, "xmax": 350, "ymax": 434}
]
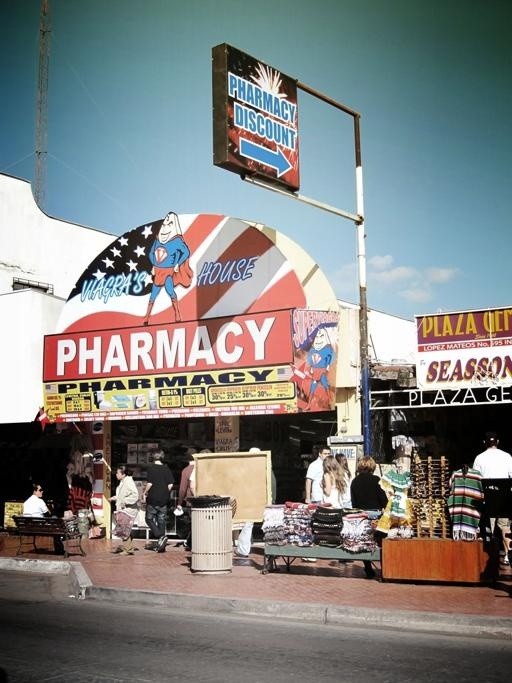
[{"xmin": 119, "ymin": 543, "xmax": 135, "ymax": 556}]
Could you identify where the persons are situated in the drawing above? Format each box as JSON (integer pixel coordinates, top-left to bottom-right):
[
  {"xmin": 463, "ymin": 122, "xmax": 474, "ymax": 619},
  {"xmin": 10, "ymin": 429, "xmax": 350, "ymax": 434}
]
[
  {"xmin": 323, "ymin": 456, "xmax": 345, "ymax": 508},
  {"xmin": 305, "ymin": 445, "xmax": 332, "ymax": 505},
  {"xmin": 474, "ymin": 432, "xmax": 512, "ymax": 502},
  {"xmin": 193, "ymin": 449, "xmax": 212, "ymax": 497},
  {"xmin": 179, "ymin": 448, "xmax": 199, "ymax": 548},
  {"xmin": 338, "ymin": 454, "xmax": 352, "ymax": 509},
  {"xmin": 108, "ymin": 465, "xmax": 138, "ymax": 553},
  {"xmin": 143, "ymin": 448, "xmax": 176, "ymax": 553},
  {"xmin": 351, "ymin": 456, "xmax": 387, "ymax": 578},
  {"xmin": 251, "ymin": 447, "xmax": 277, "ymax": 504},
  {"xmin": 22, "ymin": 483, "xmax": 67, "ymax": 556}
]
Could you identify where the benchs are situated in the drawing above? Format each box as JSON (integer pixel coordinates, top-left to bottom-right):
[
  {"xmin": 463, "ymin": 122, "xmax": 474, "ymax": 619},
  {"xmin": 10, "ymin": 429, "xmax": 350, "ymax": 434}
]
[{"xmin": 9, "ymin": 514, "xmax": 88, "ymax": 560}]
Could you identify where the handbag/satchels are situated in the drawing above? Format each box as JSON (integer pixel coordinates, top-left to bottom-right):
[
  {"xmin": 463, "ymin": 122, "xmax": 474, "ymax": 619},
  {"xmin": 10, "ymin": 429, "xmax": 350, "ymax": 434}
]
[{"xmin": 111, "ymin": 510, "xmax": 136, "ymax": 541}]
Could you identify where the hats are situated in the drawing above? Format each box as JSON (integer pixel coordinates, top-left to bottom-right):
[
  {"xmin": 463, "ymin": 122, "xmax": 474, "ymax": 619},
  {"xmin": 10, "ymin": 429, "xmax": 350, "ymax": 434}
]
[
  {"xmin": 483, "ymin": 431, "xmax": 499, "ymax": 441},
  {"xmin": 61, "ymin": 509, "xmax": 90, "ymax": 540},
  {"xmin": 184, "ymin": 448, "xmax": 196, "ymax": 455}
]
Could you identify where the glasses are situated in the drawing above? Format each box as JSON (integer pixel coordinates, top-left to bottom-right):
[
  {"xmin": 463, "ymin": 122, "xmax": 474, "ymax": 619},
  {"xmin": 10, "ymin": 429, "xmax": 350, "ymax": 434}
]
[
  {"xmin": 322, "ymin": 453, "xmax": 331, "ymax": 455},
  {"xmin": 38, "ymin": 488, "xmax": 43, "ymax": 492},
  {"xmin": 408, "ymin": 460, "xmax": 450, "ymax": 525}
]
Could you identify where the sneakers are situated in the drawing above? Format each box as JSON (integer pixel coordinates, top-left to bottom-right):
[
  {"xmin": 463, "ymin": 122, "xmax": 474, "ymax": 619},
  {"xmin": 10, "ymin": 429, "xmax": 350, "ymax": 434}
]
[{"xmin": 154, "ymin": 535, "xmax": 168, "ymax": 553}]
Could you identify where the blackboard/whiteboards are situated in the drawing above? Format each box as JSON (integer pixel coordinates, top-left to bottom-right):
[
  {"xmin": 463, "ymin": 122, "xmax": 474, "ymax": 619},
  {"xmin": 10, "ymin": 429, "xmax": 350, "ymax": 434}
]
[{"xmin": 192, "ymin": 451, "xmax": 272, "ymax": 523}]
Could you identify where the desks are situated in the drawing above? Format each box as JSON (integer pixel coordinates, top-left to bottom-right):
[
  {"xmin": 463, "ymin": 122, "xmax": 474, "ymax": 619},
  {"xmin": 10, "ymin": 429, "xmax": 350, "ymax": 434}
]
[{"xmin": 263, "ymin": 545, "xmax": 380, "ymax": 580}]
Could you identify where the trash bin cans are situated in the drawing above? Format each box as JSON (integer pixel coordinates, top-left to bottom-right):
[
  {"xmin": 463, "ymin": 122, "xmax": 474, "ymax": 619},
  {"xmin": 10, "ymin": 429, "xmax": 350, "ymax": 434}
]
[{"xmin": 186, "ymin": 496, "xmax": 233, "ymax": 575}]
